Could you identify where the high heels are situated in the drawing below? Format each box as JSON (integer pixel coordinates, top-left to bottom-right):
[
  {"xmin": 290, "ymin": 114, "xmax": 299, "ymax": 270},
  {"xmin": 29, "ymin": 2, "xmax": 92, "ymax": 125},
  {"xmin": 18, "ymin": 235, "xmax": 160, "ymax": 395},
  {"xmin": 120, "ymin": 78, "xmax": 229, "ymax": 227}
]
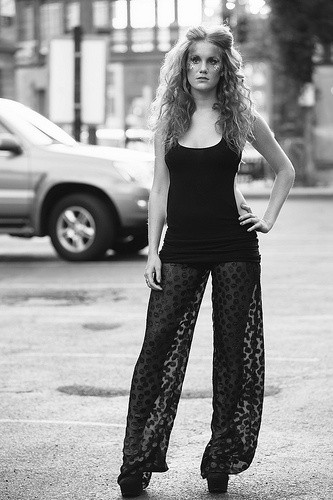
[
  {"xmin": 207, "ymin": 472, "xmax": 229, "ymax": 494},
  {"xmin": 118, "ymin": 474, "xmax": 143, "ymax": 498}
]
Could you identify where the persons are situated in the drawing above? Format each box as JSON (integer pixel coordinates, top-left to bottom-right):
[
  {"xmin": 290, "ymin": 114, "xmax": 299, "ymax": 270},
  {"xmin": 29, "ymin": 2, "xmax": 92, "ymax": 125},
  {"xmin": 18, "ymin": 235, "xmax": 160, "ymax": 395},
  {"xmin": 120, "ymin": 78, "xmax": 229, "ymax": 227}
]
[{"xmin": 116, "ymin": 24, "xmax": 296, "ymax": 500}]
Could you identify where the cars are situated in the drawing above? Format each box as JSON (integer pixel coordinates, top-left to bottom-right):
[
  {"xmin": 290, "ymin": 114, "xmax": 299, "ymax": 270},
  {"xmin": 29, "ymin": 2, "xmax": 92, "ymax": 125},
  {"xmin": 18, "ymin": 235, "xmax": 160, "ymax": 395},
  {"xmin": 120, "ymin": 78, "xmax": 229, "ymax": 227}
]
[{"xmin": 0, "ymin": 98, "xmax": 156, "ymax": 263}]
